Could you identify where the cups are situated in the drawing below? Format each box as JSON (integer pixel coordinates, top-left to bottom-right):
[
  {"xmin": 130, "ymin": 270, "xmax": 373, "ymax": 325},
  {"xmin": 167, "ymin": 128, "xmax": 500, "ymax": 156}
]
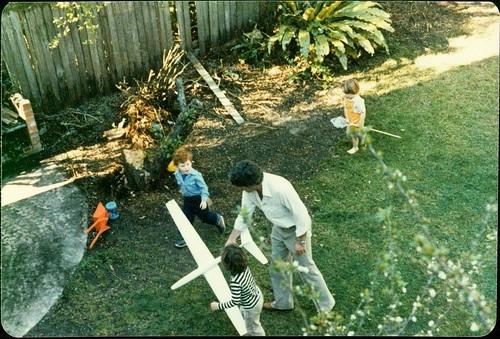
[{"xmin": 105, "ymin": 201, "xmax": 119, "ymax": 220}]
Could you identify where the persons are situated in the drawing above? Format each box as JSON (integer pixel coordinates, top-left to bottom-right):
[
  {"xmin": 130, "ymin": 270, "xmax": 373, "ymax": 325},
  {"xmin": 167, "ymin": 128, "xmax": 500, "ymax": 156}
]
[
  {"xmin": 210, "ymin": 244, "xmax": 266, "ymax": 336},
  {"xmin": 173, "ymin": 149, "xmax": 226, "ymax": 249},
  {"xmin": 224, "ymin": 159, "xmax": 335, "ymax": 325},
  {"xmin": 342, "ymin": 79, "xmax": 366, "ymax": 155}
]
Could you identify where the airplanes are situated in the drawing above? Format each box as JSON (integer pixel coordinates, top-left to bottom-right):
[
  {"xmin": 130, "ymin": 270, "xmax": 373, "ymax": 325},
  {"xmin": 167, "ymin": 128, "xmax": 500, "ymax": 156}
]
[{"xmin": 164, "ymin": 198, "xmax": 269, "ymax": 336}]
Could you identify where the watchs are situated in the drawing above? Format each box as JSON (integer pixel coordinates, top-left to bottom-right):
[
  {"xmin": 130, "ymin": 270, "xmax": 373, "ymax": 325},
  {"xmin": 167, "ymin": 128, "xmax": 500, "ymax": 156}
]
[{"xmin": 297, "ymin": 240, "xmax": 306, "ymax": 245}]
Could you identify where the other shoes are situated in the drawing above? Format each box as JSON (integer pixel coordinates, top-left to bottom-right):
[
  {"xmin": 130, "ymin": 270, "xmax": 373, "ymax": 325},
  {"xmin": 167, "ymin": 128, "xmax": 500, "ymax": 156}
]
[
  {"xmin": 217, "ymin": 216, "xmax": 226, "ymax": 234},
  {"xmin": 175, "ymin": 239, "xmax": 187, "ymax": 248},
  {"xmin": 347, "ymin": 147, "xmax": 358, "ymax": 154},
  {"xmin": 264, "ymin": 302, "xmax": 275, "ymax": 309}
]
